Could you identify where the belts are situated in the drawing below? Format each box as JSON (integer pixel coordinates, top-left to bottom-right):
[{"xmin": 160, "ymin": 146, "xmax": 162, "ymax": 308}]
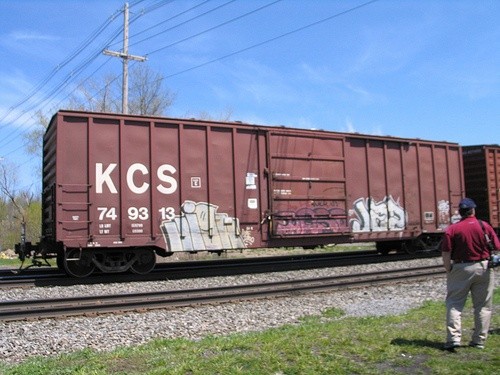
[{"xmin": 454, "ymin": 259, "xmax": 487, "ymax": 263}]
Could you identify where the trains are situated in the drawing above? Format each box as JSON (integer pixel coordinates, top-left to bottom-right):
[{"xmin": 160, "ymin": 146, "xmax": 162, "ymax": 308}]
[{"xmin": 13, "ymin": 107, "xmax": 499, "ymax": 282}]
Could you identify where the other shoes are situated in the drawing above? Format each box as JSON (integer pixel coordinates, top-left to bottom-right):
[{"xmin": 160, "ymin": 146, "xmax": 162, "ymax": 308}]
[
  {"xmin": 441, "ymin": 345, "xmax": 458, "ymax": 353},
  {"xmin": 470, "ymin": 341, "xmax": 485, "ymax": 349}
]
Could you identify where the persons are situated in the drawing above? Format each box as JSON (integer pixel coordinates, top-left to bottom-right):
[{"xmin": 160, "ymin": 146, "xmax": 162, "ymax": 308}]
[{"xmin": 442, "ymin": 198, "xmax": 500, "ymax": 348}]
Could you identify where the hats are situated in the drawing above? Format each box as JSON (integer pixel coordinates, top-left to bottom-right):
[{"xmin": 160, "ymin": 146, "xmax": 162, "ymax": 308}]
[{"xmin": 459, "ymin": 197, "xmax": 476, "ymax": 209}]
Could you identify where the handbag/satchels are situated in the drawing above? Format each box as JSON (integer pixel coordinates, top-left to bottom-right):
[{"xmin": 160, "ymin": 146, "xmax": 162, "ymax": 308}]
[{"xmin": 488, "ymin": 252, "xmax": 500, "ymax": 268}]
